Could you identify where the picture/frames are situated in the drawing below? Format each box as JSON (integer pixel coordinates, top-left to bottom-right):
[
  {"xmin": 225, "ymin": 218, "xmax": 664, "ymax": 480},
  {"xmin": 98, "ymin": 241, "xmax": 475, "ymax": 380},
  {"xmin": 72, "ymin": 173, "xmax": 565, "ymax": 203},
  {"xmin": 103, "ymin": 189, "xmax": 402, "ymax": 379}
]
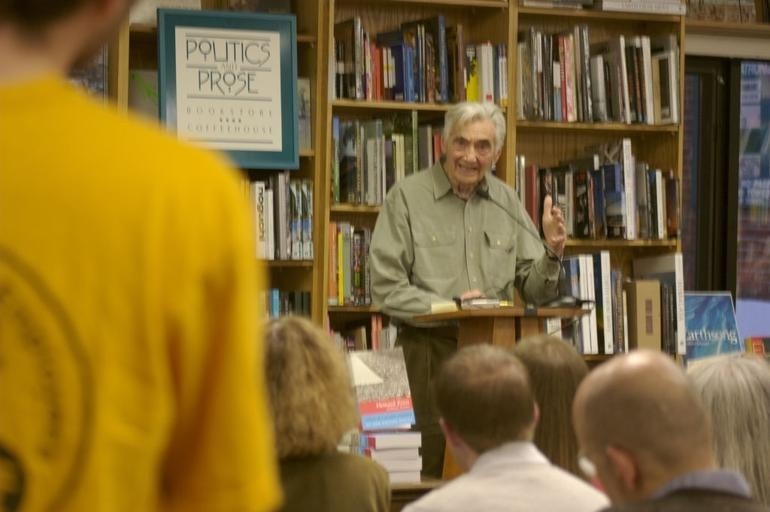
[{"xmin": 154, "ymin": 7, "xmax": 301, "ymax": 173}]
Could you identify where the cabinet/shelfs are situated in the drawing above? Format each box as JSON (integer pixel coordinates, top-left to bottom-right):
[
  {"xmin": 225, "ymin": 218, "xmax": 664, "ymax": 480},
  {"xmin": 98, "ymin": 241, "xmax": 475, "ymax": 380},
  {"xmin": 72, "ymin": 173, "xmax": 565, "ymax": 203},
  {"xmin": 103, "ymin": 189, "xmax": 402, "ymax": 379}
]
[
  {"xmin": 510, "ymin": 1, "xmax": 683, "ymax": 360},
  {"xmin": 322, "ymin": 0, "xmax": 511, "ymax": 317},
  {"xmin": 126, "ymin": 0, "xmax": 320, "ymax": 276}
]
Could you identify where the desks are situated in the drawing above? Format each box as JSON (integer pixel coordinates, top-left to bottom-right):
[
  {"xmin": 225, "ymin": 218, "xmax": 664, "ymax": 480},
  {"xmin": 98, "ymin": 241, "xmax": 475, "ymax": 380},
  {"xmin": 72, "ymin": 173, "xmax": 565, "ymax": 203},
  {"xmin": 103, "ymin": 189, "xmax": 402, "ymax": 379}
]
[{"xmin": 384, "ymin": 478, "xmax": 443, "ymax": 512}]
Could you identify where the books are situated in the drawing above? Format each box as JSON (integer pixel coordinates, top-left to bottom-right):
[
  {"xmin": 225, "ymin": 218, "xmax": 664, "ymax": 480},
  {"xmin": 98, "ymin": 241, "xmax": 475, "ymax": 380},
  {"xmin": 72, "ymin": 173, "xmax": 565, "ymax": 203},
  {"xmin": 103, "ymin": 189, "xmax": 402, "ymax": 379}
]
[
  {"xmin": 249, "ymin": 180, "xmax": 268, "ymax": 260},
  {"xmin": 585, "ymin": 255, "xmax": 599, "ymax": 355},
  {"xmin": 297, "ymin": 76, "xmax": 313, "ymax": 154},
  {"xmin": 292, "ymin": 290, "xmax": 303, "ymax": 317},
  {"xmin": 332, "ymin": 117, "xmax": 367, "ymax": 206},
  {"xmin": 367, "ymin": 110, "xmax": 443, "ymax": 207},
  {"xmin": 522, "ymin": 0, "xmax": 583, "ymax": 10},
  {"xmin": 544, "ymin": 288, "xmax": 562, "ymax": 342},
  {"xmin": 431, "ymin": 299, "xmax": 512, "ymax": 313},
  {"xmin": 515, "ymin": 24, "xmax": 688, "ymax": 127},
  {"xmin": 573, "ymin": 255, "xmax": 591, "ymax": 354},
  {"xmin": 558, "ymin": 256, "xmax": 582, "ymax": 354},
  {"xmin": 361, "ymin": 448, "xmax": 421, "ymax": 460},
  {"xmin": 596, "ymin": 0, "xmax": 686, "ymax": 16},
  {"xmin": 378, "ymin": 457, "xmax": 423, "ymax": 471},
  {"xmin": 268, "ymin": 172, "xmax": 291, "ymax": 260},
  {"xmin": 267, "ymin": 288, "xmax": 281, "ymax": 323},
  {"xmin": 262, "ymin": 189, "xmax": 275, "ymax": 261},
  {"xmin": 548, "ymin": 0, "xmax": 595, "ymax": 6},
  {"xmin": 349, "ymin": 346, "xmax": 416, "ymax": 433},
  {"xmin": 282, "ymin": 291, "xmax": 293, "ymax": 317},
  {"xmin": 593, "ymin": 250, "xmax": 613, "ymax": 355},
  {"xmin": 569, "ymin": 137, "xmax": 682, "ymax": 241},
  {"xmin": 367, "ymin": 313, "xmax": 392, "ymax": 350},
  {"xmin": 516, "ymin": 155, "xmax": 569, "ymax": 242},
  {"xmin": 351, "ymin": 431, "xmax": 422, "ymax": 451},
  {"xmin": 300, "ymin": 291, "xmax": 311, "ymax": 316},
  {"xmin": 335, "ymin": 14, "xmax": 509, "ymax": 106},
  {"xmin": 329, "ymin": 326, "xmax": 367, "ymax": 350},
  {"xmin": 614, "ymin": 250, "xmax": 688, "ymax": 355},
  {"xmin": 744, "ymin": 336, "xmax": 770, "ymax": 359},
  {"xmin": 299, "ymin": 179, "xmax": 313, "ymax": 260},
  {"xmin": 389, "ymin": 470, "xmax": 421, "ymax": 484},
  {"xmin": 328, "ymin": 222, "xmax": 367, "ymax": 307},
  {"xmin": 288, "ymin": 178, "xmax": 303, "ymax": 260}
]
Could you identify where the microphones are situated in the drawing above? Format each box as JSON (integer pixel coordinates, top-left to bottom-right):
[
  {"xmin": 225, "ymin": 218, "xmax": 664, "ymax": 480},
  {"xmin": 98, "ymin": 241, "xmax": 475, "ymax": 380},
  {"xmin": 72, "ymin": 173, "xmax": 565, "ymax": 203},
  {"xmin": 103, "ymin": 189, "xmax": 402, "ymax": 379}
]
[{"xmin": 475, "ymin": 187, "xmax": 581, "ymax": 308}]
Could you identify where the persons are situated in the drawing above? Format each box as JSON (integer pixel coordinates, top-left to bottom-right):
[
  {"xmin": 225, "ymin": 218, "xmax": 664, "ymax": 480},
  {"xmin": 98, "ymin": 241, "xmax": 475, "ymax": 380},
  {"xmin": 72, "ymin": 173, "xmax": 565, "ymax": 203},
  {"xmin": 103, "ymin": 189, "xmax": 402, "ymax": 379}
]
[
  {"xmin": 510, "ymin": 333, "xmax": 607, "ymax": 493},
  {"xmin": 685, "ymin": 350, "xmax": 770, "ymax": 512},
  {"xmin": 399, "ymin": 343, "xmax": 611, "ymax": 511},
  {"xmin": 263, "ymin": 314, "xmax": 393, "ymax": 511},
  {"xmin": 0, "ymin": 0, "xmax": 287, "ymax": 512},
  {"xmin": 570, "ymin": 347, "xmax": 763, "ymax": 512},
  {"xmin": 367, "ymin": 100, "xmax": 569, "ymax": 483}
]
